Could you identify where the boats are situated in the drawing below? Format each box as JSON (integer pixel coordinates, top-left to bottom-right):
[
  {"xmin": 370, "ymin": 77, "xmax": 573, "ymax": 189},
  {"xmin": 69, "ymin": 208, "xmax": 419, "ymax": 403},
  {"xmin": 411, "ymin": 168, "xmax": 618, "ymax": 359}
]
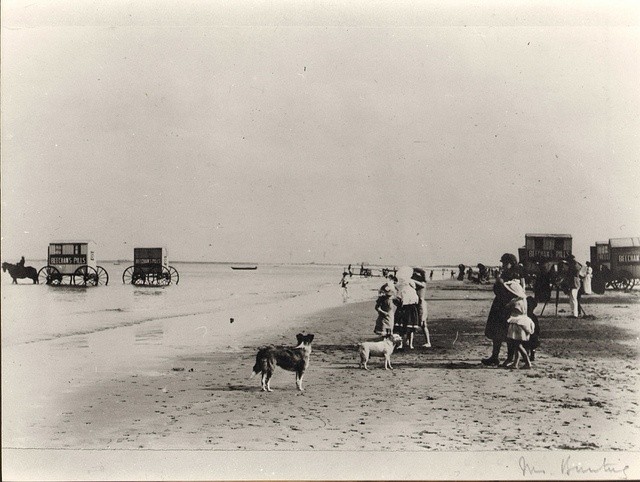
[{"xmin": 230, "ymin": 266, "xmax": 258, "ymax": 270}]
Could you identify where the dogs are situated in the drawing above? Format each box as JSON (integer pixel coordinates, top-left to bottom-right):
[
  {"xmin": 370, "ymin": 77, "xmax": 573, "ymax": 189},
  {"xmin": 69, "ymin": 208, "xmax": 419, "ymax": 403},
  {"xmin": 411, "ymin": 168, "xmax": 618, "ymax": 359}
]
[
  {"xmin": 250, "ymin": 333, "xmax": 314, "ymax": 392},
  {"xmin": 358, "ymin": 334, "xmax": 402, "ymax": 370}
]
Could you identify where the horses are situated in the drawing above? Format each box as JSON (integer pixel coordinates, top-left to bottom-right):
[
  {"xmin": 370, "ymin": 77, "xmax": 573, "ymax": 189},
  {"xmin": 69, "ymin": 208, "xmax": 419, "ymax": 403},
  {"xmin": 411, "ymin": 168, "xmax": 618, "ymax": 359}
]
[{"xmin": 2, "ymin": 262, "xmax": 38, "ymax": 285}]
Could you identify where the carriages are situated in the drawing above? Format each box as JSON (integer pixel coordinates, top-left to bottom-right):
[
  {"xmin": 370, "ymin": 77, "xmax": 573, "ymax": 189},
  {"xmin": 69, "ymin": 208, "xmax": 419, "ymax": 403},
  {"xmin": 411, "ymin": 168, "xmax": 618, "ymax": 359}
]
[
  {"xmin": 607, "ymin": 236, "xmax": 640, "ymax": 291},
  {"xmin": 120, "ymin": 246, "xmax": 180, "ymax": 287},
  {"xmin": 2, "ymin": 240, "xmax": 108, "ymax": 286},
  {"xmin": 583, "ymin": 242, "xmax": 610, "ymax": 294},
  {"xmin": 523, "ymin": 232, "xmax": 575, "ymax": 294}
]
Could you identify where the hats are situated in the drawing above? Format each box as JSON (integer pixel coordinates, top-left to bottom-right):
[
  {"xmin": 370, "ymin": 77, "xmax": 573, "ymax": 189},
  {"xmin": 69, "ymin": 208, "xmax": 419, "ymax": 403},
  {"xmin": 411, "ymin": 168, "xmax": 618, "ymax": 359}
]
[
  {"xmin": 503, "ymin": 281, "xmax": 526, "ymax": 297},
  {"xmin": 499, "ymin": 253, "xmax": 517, "ymax": 263}
]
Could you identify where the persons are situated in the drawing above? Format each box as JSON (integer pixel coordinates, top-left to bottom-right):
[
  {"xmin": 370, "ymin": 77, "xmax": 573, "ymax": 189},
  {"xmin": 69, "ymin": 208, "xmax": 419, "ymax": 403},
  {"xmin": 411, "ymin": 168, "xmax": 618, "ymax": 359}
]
[
  {"xmin": 480, "ymin": 252, "xmax": 525, "ymax": 369},
  {"xmin": 373, "ymin": 281, "xmax": 397, "ymax": 342},
  {"xmin": 583, "ymin": 260, "xmax": 594, "ymax": 295},
  {"xmin": 339, "ymin": 272, "xmax": 351, "ymax": 298},
  {"xmin": 405, "ymin": 267, "xmax": 432, "ymax": 349},
  {"xmin": 393, "ymin": 263, "xmax": 420, "ymax": 351},
  {"xmin": 502, "ymin": 280, "xmax": 535, "ymax": 371},
  {"xmin": 525, "ymin": 296, "xmax": 540, "ymax": 361},
  {"xmin": 559, "ymin": 253, "xmax": 583, "ymax": 319}
]
